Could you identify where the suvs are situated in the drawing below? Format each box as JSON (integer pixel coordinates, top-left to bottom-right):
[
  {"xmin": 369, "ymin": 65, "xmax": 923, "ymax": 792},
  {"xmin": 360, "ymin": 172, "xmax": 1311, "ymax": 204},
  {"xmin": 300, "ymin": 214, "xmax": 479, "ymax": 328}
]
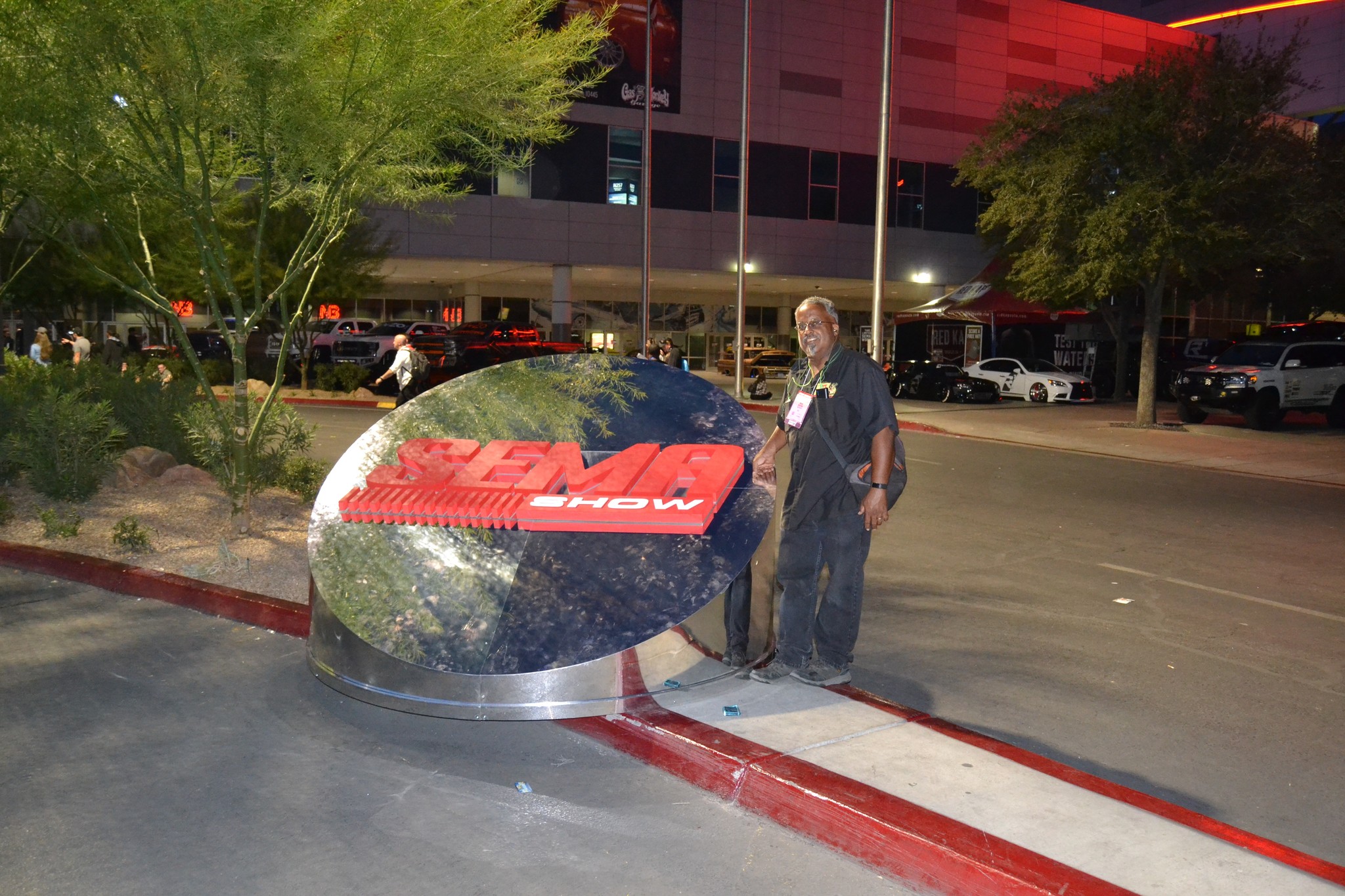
[
  {"xmin": 1093, "ymin": 319, "xmax": 1344, "ymax": 431},
  {"xmin": 186, "ymin": 316, "xmax": 282, "ymax": 361},
  {"xmin": 264, "ymin": 317, "xmax": 379, "ymax": 373},
  {"xmin": 330, "ymin": 320, "xmax": 451, "ymax": 378}
]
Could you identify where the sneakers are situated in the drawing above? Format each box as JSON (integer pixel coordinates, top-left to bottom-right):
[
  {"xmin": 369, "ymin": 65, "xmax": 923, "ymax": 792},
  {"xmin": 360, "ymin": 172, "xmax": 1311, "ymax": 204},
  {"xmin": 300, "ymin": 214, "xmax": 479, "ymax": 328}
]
[
  {"xmin": 750, "ymin": 653, "xmax": 809, "ymax": 685},
  {"xmin": 722, "ymin": 651, "xmax": 753, "ymax": 679},
  {"xmin": 787, "ymin": 659, "xmax": 852, "ymax": 688}
]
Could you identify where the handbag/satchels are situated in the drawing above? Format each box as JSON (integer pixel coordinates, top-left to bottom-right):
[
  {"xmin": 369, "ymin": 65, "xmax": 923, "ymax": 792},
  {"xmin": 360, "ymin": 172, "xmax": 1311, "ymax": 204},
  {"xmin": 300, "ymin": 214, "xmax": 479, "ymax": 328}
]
[{"xmin": 847, "ymin": 429, "xmax": 907, "ymax": 521}]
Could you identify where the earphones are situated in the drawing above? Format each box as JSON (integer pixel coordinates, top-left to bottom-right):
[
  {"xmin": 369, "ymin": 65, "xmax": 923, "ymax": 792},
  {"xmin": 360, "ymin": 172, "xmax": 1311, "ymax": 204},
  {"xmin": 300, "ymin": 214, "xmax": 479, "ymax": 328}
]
[{"xmin": 833, "ymin": 328, "xmax": 837, "ymax": 332}]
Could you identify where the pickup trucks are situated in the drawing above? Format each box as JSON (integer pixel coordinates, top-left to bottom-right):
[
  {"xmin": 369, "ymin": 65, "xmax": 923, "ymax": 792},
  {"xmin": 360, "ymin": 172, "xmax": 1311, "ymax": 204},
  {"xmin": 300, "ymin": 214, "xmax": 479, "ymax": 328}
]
[
  {"xmin": 717, "ymin": 346, "xmax": 795, "ymax": 379},
  {"xmin": 409, "ymin": 320, "xmax": 585, "ymax": 381}
]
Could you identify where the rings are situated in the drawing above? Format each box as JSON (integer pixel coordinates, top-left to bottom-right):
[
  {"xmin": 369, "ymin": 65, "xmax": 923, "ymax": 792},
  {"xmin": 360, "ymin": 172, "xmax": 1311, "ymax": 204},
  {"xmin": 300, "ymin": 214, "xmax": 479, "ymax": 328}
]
[{"xmin": 877, "ymin": 517, "xmax": 883, "ymax": 520}]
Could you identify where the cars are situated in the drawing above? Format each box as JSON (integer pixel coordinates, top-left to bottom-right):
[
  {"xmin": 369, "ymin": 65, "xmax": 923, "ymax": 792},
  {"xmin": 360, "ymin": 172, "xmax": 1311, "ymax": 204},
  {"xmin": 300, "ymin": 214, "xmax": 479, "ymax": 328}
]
[{"xmin": 881, "ymin": 357, "xmax": 1096, "ymax": 405}]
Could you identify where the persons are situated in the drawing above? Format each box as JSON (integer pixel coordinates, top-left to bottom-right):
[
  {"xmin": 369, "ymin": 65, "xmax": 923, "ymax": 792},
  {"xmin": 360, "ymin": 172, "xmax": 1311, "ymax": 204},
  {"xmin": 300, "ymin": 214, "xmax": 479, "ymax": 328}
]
[
  {"xmin": 64, "ymin": 329, "xmax": 74, "ymax": 349},
  {"xmin": 4, "ymin": 332, "xmax": 14, "ymax": 352},
  {"xmin": 61, "ymin": 326, "xmax": 92, "ymax": 374},
  {"xmin": 750, "ymin": 373, "xmax": 773, "ymax": 401},
  {"xmin": 375, "ymin": 334, "xmax": 417, "ymax": 406},
  {"xmin": 750, "ymin": 295, "xmax": 901, "ymax": 687},
  {"xmin": 147, "ymin": 362, "xmax": 175, "ymax": 392},
  {"xmin": 128, "ymin": 327, "xmax": 147, "ymax": 353},
  {"xmin": 101, "ymin": 327, "xmax": 124, "ymax": 378},
  {"xmin": 720, "ymin": 558, "xmax": 755, "ymax": 674},
  {"xmin": 114, "ymin": 333, "xmax": 127, "ymax": 377},
  {"xmin": 29, "ymin": 325, "xmax": 54, "ymax": 375},
  {"xmin": 662, "ymin": 337, "xmax": 682, "ymax": 370},
  {"xmin": 402, "ymin": 333, "xmax": 425, "ymax": 395},
  {"xmin": 647, "ymin": 343, "xmax": 668, "ymax": 365}
]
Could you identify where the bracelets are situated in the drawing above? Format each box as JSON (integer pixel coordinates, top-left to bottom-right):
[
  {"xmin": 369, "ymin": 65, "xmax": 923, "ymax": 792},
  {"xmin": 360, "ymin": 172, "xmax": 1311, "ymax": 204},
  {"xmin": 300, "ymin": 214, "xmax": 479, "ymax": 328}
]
[
  {"xmin": 869, "ymin": 482, "xmax": 888, "ymax": 490},
  {"xmin": 68, "ymin": 340, "xmax": 71, "ymax": 343},
  {"xmin": 379, "ymin": 376, "xmax": 384, "ymax": 381}
]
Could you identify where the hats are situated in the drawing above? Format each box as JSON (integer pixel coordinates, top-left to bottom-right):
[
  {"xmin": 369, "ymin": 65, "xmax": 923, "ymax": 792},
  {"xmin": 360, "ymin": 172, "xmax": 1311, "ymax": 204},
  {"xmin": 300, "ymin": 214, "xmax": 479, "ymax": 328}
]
[
  {"xmin": 68, "ymin": 326, "xmax": 83, "ymax": 336},
  {"xmin": 34, "ymin": 326, "xmax": 48, "ymax": 334},
  {"xmin": 658, "ymin": 336, "xmax": 672, "ymax": 348}
]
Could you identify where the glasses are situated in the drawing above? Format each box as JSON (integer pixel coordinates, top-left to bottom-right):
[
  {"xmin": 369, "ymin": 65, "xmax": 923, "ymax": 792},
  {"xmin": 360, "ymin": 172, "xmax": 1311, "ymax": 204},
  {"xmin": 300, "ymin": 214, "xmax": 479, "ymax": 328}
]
[{"xmin": 794, "ymin": 318, "xmax": 834, "ymax": 332}]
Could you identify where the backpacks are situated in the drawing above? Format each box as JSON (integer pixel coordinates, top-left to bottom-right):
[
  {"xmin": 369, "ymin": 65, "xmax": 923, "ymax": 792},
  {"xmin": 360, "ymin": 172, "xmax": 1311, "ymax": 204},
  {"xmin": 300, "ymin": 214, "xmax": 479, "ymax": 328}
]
[
  {"xmin": 401, "ymin": 348, "xmax": 430, "ymax": 383},
  {"xmin": 747, "ymin": 383, "xmax": 757, "ymax": 393}
]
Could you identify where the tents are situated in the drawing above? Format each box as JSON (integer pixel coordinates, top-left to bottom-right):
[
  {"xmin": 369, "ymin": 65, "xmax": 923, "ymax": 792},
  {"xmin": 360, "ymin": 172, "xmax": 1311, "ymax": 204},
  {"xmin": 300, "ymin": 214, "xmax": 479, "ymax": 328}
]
[{"xmin": 891, "ymin": 259, "xmax": 1098, "ymax": 376}]
